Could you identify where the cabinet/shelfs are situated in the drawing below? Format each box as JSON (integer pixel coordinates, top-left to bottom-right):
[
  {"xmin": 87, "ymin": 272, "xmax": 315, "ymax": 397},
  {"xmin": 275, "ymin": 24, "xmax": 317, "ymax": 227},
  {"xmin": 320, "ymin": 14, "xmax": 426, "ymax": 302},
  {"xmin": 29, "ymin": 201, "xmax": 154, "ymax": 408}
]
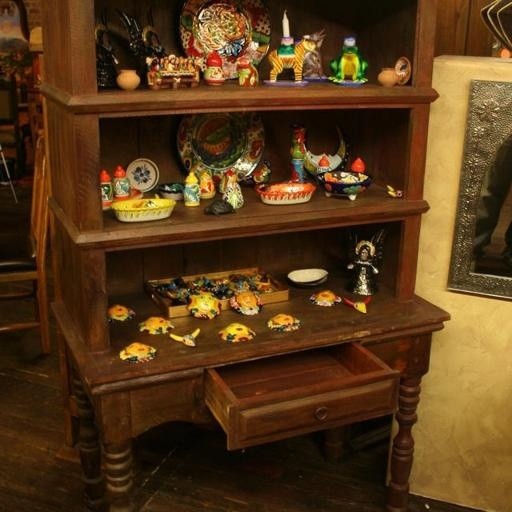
[
  {"xmin": 445, "ymin": 79, "xmax": 512, "ymax": 303},
  {"xmin": 38, "ymin": 0, "xmax": 450, "ymax": 511}
]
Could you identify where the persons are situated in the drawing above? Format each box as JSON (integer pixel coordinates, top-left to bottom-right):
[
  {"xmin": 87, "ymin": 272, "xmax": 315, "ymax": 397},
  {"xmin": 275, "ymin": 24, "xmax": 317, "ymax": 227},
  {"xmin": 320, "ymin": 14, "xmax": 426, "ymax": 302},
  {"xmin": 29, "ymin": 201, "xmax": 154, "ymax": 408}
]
[
  {"xmin": 93, "ymin": 23, "xmax": 119, "ymax": 88},
  {"xmin": 348, "ymin": 238, "xmax": 378, "ymax": 295},
  {"xmin": 143, "ymin": 25, "xmax": 167, "ymax": 62}
]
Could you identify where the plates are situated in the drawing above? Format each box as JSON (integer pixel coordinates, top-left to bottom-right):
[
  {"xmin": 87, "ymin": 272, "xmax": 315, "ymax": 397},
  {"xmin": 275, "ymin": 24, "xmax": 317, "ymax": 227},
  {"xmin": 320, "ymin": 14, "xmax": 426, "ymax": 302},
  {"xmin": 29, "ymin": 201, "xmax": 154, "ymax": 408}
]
[
  {"xmin": 125, "ymin": 157, "xmax": 160, "ymax": 193},
  {"xmin": 158, "ymin": 182, "xmax": 185, "ymax": 201},
  {"xmin": 175, "ymin": 110, "xmax": 268, "ymax": 185},
  {"xmin": 286, "ymin": 267, "xmax": 330, "ymax": 287},
  {"xmin": 179, "ymin": 0, "xmax": 273, "ymax": 80}
]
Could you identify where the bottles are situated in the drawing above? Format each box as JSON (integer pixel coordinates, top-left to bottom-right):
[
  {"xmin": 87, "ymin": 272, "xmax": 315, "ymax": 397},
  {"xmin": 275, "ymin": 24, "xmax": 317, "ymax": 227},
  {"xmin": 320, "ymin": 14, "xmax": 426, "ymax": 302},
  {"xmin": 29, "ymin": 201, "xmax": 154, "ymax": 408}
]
[{"xmin": 100, "ymin": 162, "xmax": 127, "ymax": 210}]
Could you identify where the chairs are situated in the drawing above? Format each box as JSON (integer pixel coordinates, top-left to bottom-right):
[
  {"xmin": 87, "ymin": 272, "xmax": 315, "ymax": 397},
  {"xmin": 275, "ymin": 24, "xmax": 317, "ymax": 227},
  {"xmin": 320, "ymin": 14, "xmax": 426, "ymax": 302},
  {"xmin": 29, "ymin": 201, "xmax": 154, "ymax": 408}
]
[{"xmin": 1, "ymin": 131, "xmax": 53, "ymax": 356}]
